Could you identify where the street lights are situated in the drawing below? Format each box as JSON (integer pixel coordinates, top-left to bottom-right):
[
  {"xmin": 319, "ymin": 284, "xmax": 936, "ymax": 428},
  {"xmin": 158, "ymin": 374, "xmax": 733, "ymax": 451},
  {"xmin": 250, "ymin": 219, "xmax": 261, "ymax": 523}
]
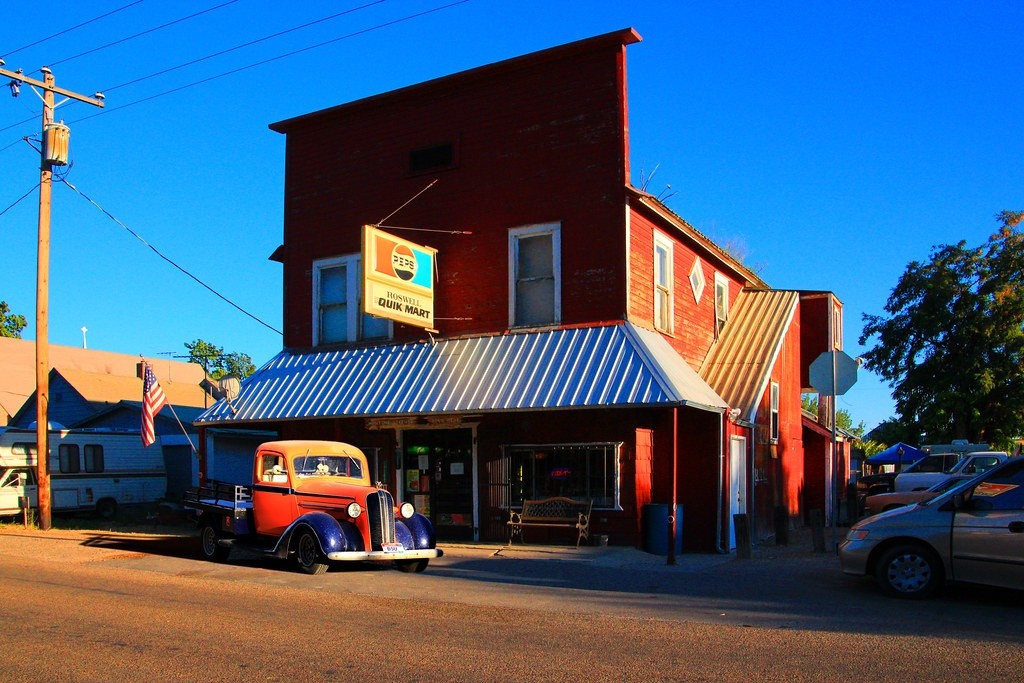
[{"xmin": 80, "ymin": 326, "xmax": 88, "ymax": 349}]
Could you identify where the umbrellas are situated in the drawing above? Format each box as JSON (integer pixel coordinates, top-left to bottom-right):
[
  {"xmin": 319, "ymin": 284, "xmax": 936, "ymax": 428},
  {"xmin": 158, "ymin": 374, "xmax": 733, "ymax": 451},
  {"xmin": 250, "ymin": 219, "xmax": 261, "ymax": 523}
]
[{"xmin": 866, "ymin": 442, "xmax": 928, "ymax": 472}]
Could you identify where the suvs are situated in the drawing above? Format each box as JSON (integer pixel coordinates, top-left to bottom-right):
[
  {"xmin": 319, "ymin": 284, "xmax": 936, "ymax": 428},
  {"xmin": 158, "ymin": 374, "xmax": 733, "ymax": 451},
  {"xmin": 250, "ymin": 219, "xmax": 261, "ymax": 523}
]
[
  {"xmin": 834, "ymin": 455, "xmax": 1024, "ymax": 603},
  {"xmin": 854, "ymin": 450, "xmax": 1009, "ymax": 517}
]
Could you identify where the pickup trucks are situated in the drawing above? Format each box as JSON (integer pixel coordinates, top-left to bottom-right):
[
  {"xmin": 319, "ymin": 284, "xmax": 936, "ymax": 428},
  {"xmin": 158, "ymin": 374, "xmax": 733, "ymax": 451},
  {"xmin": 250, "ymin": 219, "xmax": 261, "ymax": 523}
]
[{"xmin": 177, "ymin": 438, "xmax": 444, "ymax": 577}]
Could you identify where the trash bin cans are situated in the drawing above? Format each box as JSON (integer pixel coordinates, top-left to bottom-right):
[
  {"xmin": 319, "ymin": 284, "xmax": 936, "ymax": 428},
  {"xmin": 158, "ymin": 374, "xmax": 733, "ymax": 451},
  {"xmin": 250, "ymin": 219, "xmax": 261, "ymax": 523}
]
[{"xmin": 645, "ymin": 503, "xmax": 683, "ymax": 555}]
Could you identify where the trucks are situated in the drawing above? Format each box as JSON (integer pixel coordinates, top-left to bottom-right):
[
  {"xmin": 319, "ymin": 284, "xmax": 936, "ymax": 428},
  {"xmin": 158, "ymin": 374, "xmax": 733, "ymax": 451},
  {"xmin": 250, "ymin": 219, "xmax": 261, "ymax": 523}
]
[{"xmin": 0, "ymin": 429, "xmax": 169, "ymax": 522}]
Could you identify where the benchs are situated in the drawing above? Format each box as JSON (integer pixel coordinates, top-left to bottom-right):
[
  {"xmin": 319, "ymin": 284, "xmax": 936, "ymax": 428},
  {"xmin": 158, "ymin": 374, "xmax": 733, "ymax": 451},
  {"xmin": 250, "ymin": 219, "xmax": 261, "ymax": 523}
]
[{"xmin": 508, "ymin": 498, "xmax": 592, "ymax": 549}]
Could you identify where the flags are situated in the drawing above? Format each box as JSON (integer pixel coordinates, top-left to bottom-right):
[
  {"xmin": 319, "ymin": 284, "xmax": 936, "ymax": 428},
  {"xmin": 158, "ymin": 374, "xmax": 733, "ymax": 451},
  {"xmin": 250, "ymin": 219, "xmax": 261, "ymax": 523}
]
[{"xmin": 140, "ymin": 362, "xmax": 167, "ymax": 448}]
